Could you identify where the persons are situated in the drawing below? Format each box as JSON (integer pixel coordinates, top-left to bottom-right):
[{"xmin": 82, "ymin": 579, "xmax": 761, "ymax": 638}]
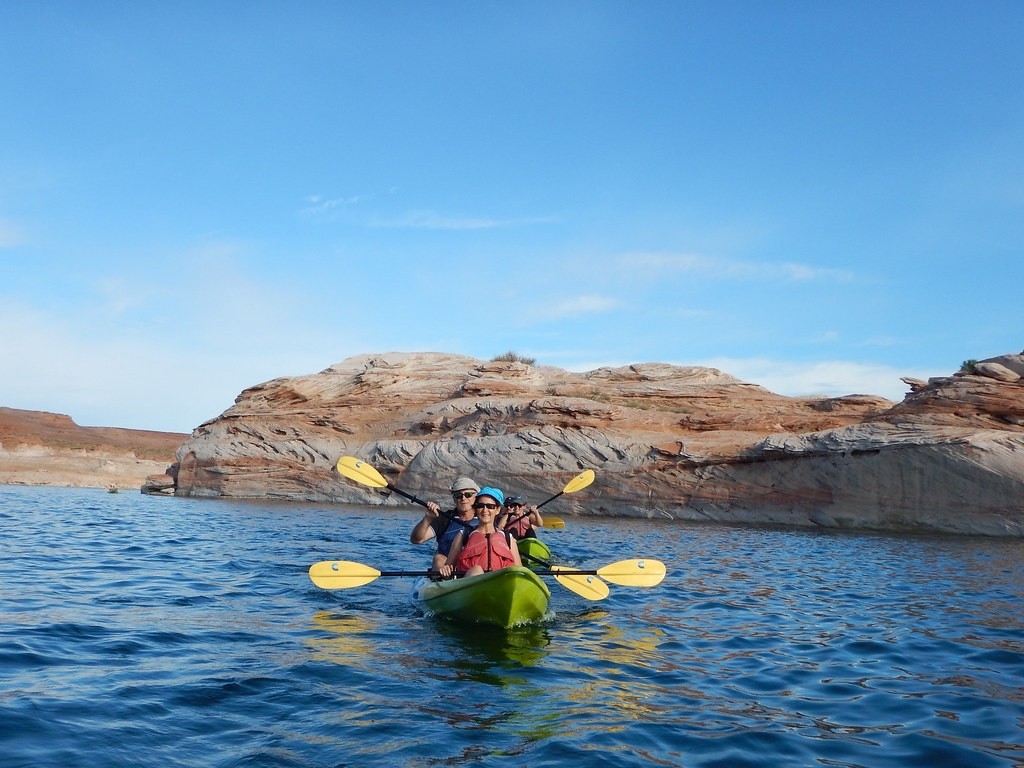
[
  {"xmin": 493, "ymin": 493, "xmax": 520, "ymax": 528},
  {"xmin": 411, "ymin": 476, "xmax": 482, "ymax": 578},
  {"xmin": 440, "ymin": 485, "xmax": 522, "ymax": 580},
  {"xmin": 499, "ymin": 498, "xmax": 544, "ymax": 541}
]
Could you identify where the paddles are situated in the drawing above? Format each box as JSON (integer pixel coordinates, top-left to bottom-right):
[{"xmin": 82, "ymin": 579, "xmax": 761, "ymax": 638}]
[
  {"xmin": 337, "ymin": 456, "xmax": 609, "ymax": 600},
  {"xmin": 309, "ymin": 559, "xmax": 666, "ymax": 589},
  {"xmin": 540, "ymin": 516, "xmax": 565, "ymax": 528},
  {"xmin": 502, "ymin": 470, "xmax": 595, "ymax": 531}
]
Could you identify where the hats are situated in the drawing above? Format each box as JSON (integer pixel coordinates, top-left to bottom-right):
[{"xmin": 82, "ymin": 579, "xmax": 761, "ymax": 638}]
[
  {"xmin": 504, "ymin": 497, "xmax": 513, "ymax": 505},
  {"xmin": 449, "ymin": 478, "xmax": 481, "ymax": 494},
  {"xmin": 513, "ymin": 495, "xmax": 528, "ymax": 505},
  {"xmin": 475, "ymin": 486, "xmax": 505, "ymax": 505}
]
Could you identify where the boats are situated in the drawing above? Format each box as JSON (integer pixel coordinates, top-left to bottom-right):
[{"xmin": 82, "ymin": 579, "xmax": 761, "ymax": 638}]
[
  {"xmin": 409, "ymin": 565, "xmax": 551, "ymax": 629},
  {"xmin": 517, "ymin": 538, "xmax": 551, "ymax": 567},
  {"xmin": 108, "ymin": 488, "xmax": 119, "ymax": 494}
]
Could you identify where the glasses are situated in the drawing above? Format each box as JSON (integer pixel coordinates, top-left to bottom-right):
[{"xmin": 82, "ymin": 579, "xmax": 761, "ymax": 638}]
[
  {"xmin": 452, "ymin": 492, "xmax": 476, "ymax": 499},
  {"xmin": 476, "ymin": 503, "xmax": 498, "ymax": 509},
  {"xmin": 513, "ymin": 503, "xmax": 525, "ymax": 508},
  {"xmin": 504, "ymin": 504, "xmax": 514, "ymax": 508}
]
